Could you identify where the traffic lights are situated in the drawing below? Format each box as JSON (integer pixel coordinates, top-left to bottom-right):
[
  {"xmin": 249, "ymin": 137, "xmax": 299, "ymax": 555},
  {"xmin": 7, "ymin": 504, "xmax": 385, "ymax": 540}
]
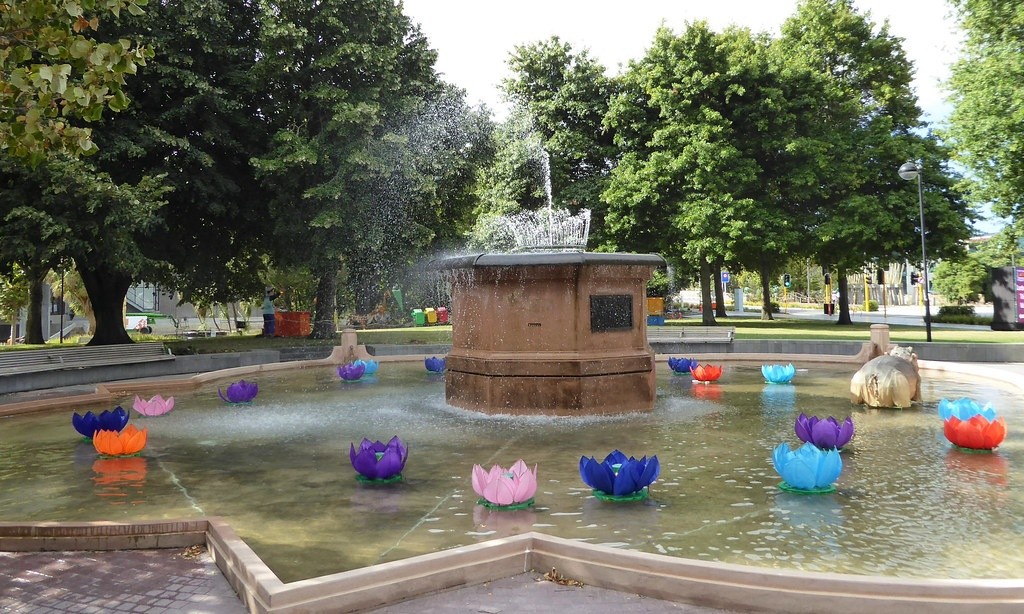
[
  {"xmin": 824, "ymin": 273, "xmax": 830, "ymax": 285},
  {"xmin": 784, "ymin": 274, "xmax": 790, "ymax": 287},
  {"xmin": 911, "ymin": 272, "xmax": 919, "ymax": 285},
  {"xmin": 866, "ymin": 276, "xmax": 872, "ymax": 285}
]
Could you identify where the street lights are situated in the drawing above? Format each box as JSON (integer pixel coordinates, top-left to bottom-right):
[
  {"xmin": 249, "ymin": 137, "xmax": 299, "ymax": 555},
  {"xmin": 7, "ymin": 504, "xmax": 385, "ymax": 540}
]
[
  {"xmin": 897, "ymin": 162, "xmax": 933, "ymax": 342},
  {"xmin": 55, "ymin": 262, "xmax": 63, "ymax": 343}
]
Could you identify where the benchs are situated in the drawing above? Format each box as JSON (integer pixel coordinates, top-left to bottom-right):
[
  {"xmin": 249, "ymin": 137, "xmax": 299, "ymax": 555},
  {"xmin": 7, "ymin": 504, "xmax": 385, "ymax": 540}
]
[
  {"xmin": 0, "ymin": 341, "xmax": 177, "ymax": 376},
  {"xmin": 646, "ymin": 325, "xmax": 735, "ymax": 344}
]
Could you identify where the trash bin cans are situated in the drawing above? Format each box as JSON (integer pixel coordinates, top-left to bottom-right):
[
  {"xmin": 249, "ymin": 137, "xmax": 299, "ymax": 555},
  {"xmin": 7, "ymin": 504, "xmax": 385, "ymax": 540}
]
[
  {"xmin": 274, "ymin": 311, "xmax": 310, "ymax": 337},
  {"xmin": 424, "ymin": 308, "xmax": 437, "ymax": 326},
  {"xmin": 411, "ymin": 308, "xmax": 425, "ymax": 327},
  {"xmin": 437, "ymin": 306, "xmax": 448, "ymax": 326},
  {"xmin": 823, "ymin": 303, "xmax": 835, "ymax": 315}
]
